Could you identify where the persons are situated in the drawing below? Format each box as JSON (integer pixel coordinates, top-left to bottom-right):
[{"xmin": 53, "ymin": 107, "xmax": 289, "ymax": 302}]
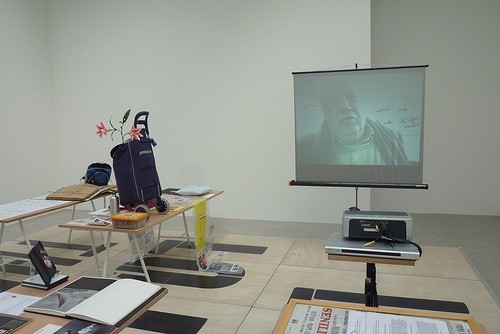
[{"xmin": 296, "ymin": 79, "xmax": 408, "ymax": 165}]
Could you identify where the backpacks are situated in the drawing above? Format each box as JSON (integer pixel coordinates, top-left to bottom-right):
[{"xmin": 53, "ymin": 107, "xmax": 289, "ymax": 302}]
[{"xmin": 80, "ymin": 162, "xmax": 112, "ymax": 186}]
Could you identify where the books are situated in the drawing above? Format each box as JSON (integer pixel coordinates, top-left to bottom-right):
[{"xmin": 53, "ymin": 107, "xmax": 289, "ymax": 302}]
[
  {"xmin": 21, "ymin": 272, "xmax": 69, "ymax": 290},
  {"xmin": 24, "ymin": 276, "xmax": 161, "ymax": 326}
]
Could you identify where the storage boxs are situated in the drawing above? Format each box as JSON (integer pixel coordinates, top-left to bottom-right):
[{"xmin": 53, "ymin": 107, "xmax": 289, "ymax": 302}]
[{"xmin": 111, "ymin": 211, "xmax": 147, "ymax": 229}]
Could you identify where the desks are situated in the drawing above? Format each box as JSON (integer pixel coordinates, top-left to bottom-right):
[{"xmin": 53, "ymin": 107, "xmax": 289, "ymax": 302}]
[{"xmin": 0, "ymin": 188, "xmax": 224, "ymax": 334}]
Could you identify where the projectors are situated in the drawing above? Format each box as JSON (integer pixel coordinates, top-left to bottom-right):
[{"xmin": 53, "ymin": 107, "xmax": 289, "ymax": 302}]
[{"xmin": 342, "ymin": 211, "xmax": 413, "ymax": 241}]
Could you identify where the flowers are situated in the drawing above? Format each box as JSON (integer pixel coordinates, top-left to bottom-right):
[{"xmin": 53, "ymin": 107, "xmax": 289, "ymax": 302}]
[{"xmin": 96, "ymin": 109, "xmax": 144, "ymax": 144}]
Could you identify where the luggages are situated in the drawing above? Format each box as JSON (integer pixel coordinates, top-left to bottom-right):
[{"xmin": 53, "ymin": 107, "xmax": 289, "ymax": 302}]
[{"xmin": 110, "ymin": 111, "xmax": 170, "ymax": 222}]
